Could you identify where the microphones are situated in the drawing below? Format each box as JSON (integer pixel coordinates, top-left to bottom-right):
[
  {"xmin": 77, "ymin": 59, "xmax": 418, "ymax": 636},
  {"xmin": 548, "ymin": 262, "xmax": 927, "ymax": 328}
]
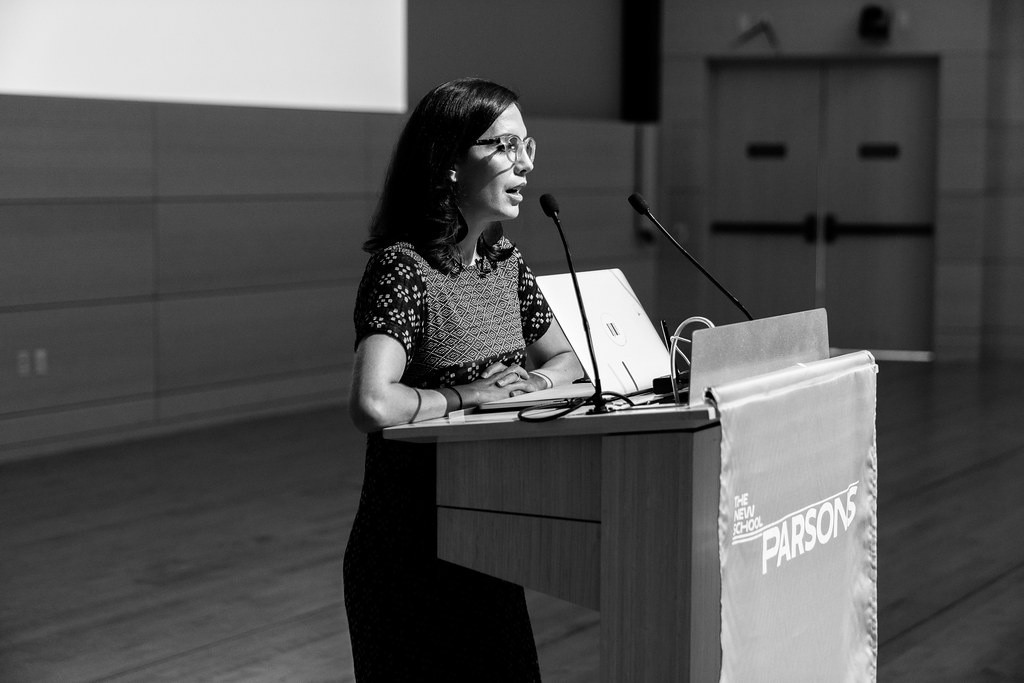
[
  {"xmin": 628, "ymin": 192, "xmax": 756, "ymax": 320},
  {"xmin": 537, "ymin": 192, "xmax": 615, "ymax": 415}
]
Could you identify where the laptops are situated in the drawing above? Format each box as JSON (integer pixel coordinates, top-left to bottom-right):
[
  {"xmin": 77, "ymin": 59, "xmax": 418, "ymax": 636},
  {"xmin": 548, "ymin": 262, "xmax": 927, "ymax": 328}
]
[{"xmin": 479, "ymin": 267, "xmax": 675, "ymax": 412}]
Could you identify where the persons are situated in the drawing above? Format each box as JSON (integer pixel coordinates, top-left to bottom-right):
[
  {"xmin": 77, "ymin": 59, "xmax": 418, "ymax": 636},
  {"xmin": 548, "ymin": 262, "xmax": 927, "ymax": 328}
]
[{"xmin": 341, "ymin": 79, "xmax": 586, "ymax": 683}]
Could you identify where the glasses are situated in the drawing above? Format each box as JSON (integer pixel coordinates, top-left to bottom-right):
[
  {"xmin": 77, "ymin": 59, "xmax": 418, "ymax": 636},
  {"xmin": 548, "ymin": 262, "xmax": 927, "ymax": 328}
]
[{"xmin": 472, "ymin": 134, "xmax": 537, "ymax": 162}]
